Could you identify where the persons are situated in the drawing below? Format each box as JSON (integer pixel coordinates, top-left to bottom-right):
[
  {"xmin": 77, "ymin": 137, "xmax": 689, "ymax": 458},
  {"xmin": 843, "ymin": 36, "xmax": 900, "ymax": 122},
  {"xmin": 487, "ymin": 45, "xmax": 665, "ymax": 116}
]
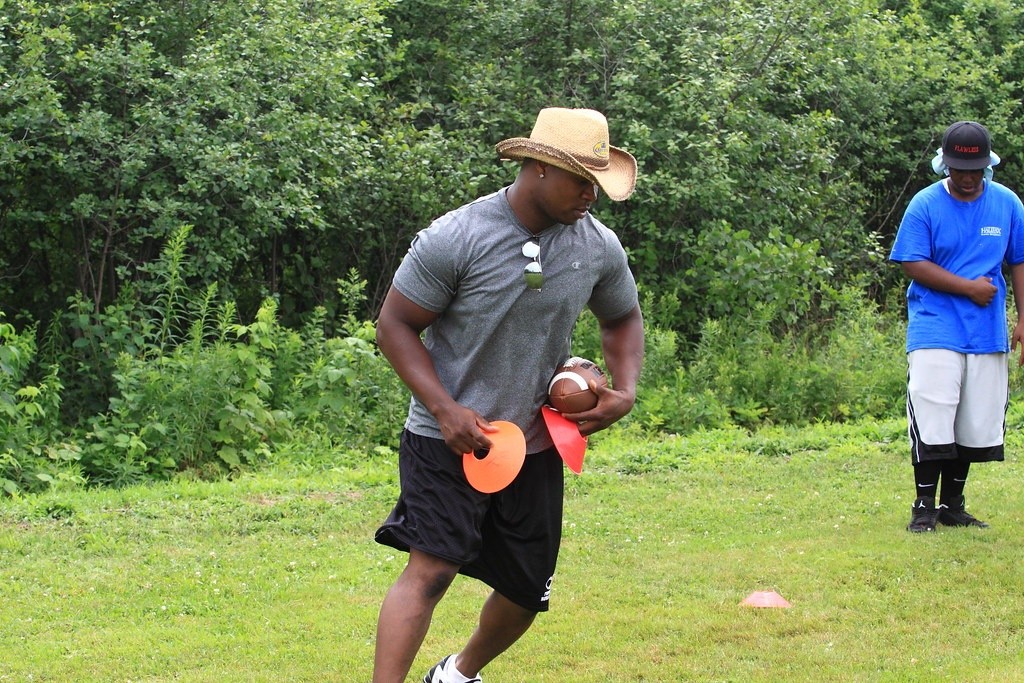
[
  {"xmin": 889, "ymin": 121, "xmax": 1023, "ymax": 532},
  {"xmin": 373, "ymin": 105, "xmax": 644, "ymax": 682}
]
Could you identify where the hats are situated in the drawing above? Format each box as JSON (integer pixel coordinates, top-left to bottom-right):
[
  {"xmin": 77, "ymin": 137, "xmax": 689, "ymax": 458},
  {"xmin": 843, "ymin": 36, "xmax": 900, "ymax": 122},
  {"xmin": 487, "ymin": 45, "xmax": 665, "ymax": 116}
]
[
  {"xmin": 495, "ymin": 107, "xmax": 637, "ymax": 202},
  {"xmin": 942, "ymin": 121, "xmax": 991, "ymax": 170}
]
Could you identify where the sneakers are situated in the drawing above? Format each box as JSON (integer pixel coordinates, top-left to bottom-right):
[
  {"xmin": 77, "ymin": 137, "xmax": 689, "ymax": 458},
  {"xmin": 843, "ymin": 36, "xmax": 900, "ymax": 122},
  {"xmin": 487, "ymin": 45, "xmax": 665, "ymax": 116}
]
[
  {"xmin": 939, "ymin": 495, "xmax": 989, "ymax": 527},
  {"xmin": 906, "ymin": 496, "xmax": 939, "ymax": 532},
  {"xmin": 424, "ymin": 654, "xmax": 482, "ymax": 683}
]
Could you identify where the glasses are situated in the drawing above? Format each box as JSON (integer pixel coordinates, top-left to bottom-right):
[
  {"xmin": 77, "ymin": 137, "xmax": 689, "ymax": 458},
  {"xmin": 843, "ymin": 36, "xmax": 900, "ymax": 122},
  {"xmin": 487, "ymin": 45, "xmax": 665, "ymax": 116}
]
[{"xmin": 522, "ymin": 235, "xmax": 544, "ymax": 293}]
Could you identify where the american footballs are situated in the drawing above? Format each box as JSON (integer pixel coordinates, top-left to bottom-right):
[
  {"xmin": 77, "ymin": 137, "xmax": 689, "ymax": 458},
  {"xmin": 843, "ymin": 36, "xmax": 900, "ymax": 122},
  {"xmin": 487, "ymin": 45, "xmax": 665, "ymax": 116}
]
[{"xmin": 547, "ymin": 355, "xmax": 611, "ymax": 416}]
